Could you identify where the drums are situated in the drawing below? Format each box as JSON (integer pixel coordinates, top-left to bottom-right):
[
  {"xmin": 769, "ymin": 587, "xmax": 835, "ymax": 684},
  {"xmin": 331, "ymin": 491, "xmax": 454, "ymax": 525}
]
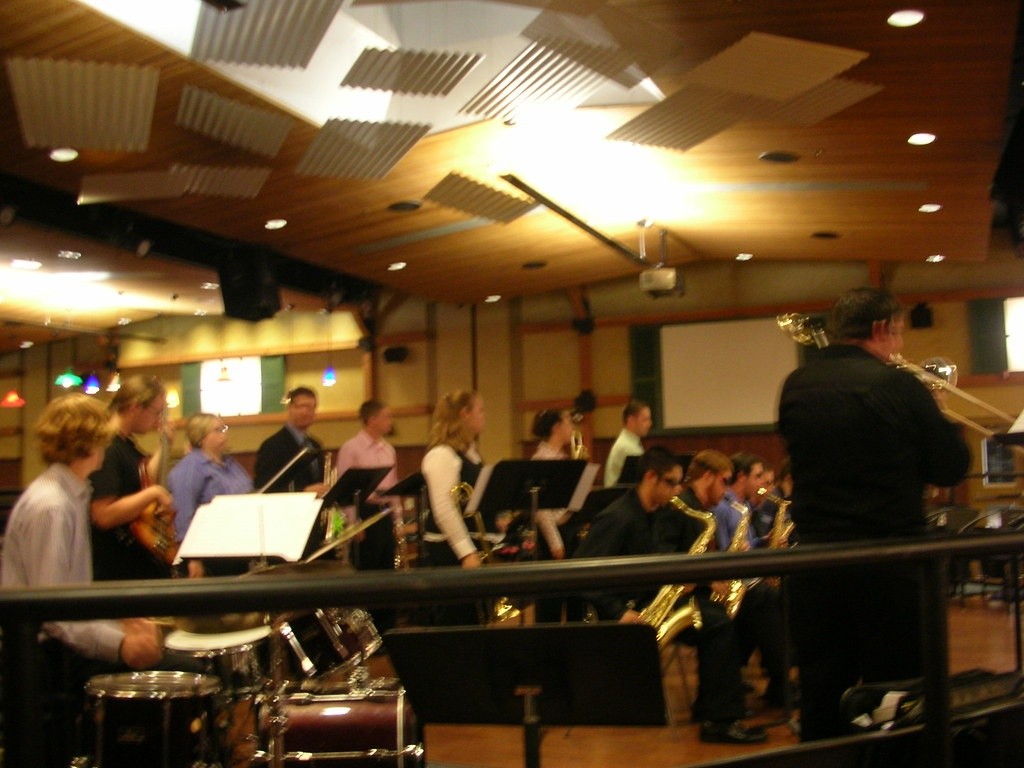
[
  {"xmin": 82, "ymin": 671, "xmax": 222, "ymax": 768},
  {"xmin": 161, "ymin": 624, "xmax": 273, "ymax": 702},
  {"xmin": 254, "ymin": 680, "xmax": 427, "ymax": 768},
  {"xmin": 270, "ymin": 607, "xmax": 383, "ymax": 681}
]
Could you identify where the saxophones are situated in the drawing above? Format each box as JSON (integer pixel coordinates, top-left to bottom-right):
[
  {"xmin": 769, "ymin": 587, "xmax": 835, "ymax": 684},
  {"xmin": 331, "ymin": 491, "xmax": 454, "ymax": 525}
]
[{"xmin": 635, "ymin": 488, "xmax": 794, "ymax": 650}]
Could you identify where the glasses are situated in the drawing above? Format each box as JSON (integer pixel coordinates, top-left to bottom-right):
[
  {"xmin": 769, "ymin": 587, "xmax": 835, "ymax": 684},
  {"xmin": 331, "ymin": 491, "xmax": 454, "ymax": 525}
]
[{"xmin": 666, "ymin": 479, "xmax": 682, "ymax": 486}]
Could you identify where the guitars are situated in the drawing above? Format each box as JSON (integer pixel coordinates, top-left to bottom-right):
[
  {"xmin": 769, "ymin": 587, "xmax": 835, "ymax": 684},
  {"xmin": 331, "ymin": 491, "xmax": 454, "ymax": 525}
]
[{"xmin": 131, "ymin": 434, "xmax": 177, "ymax": 563}]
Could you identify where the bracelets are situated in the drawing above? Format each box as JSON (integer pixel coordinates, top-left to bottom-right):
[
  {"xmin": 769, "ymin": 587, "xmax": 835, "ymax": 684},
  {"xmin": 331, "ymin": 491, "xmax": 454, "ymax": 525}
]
[{"xmin": 153, "ymin": 537, "xmax": 175, "ymax": 562}]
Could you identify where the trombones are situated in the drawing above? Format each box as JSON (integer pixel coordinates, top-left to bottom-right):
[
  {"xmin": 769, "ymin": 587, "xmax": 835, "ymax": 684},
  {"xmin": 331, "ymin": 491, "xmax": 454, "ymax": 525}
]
[
  {"xmin": 774, "ymin": 308, "xmax": 1017, "ymax": 437},
  {"xmin": 569, "ymin": 429, "xmax": 592, "ymax": 539},
  {"xmin": 450, "ymin": 482, "xmax": 523, "ymax": 626}
]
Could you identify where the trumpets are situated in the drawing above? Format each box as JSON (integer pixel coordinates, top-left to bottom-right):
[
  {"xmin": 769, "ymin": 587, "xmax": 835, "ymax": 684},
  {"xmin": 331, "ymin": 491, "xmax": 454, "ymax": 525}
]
[{"xmin": 321, "ymin": 450, "xmax": 340, "ymax": 527}]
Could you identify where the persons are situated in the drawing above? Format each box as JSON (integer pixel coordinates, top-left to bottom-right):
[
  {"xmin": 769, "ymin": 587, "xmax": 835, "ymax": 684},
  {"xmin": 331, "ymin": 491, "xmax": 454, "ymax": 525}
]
[
  {"xmin": 166, "ymin": 412, "xmax": 259, "ymax": 577},
  {"xmin": 255, "ymin": 388, "xmax": 330, "ymax": 567},
  {"xmin": 336, "ymin": 399, "xmax": 401, "ymax": 633},
  {"xmin": 775, "ymin": 284, "xmax": 973, "ymax": 768},
  {"xmin": 653, "ymin": 448, "xmax": 797, "ymax": 715},
  {"xmin": 604, "ymin": 402, "xmax": 653, "ymax": 490},
  {"xmin": 0, "ymin": 395, "xmax": 165, "ymax": 766},
  {"xmin": 89, "ymin": 374, "xmax": 176, "ymax": 581},
  {"xmin": 420, "ymin": 388, "xmax": 489, "ymax": 625},
  {"xmin": 526, "ymin": 408, "xmax": 587, "ymax": 624},
  {"xmin": 570, "ymin": 447, "xmax": 768, "ymax": 746}
]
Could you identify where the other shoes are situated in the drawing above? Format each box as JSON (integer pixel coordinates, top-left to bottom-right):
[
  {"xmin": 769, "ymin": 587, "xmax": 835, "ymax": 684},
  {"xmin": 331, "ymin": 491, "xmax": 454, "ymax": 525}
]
[
  {"xmin": 701, "ymin": 722, "xmax": 767, "ymax": 742},
  {"xmin": 692, "ymin": 709, "xmax": 750, "ymax": 722}
]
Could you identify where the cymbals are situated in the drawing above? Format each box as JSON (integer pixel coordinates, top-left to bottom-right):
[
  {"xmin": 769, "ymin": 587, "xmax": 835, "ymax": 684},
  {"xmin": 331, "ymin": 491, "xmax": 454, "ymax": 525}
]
[{"xmin": 241, "ymin": 561, "xmax": 339, "ymax": 574}]
